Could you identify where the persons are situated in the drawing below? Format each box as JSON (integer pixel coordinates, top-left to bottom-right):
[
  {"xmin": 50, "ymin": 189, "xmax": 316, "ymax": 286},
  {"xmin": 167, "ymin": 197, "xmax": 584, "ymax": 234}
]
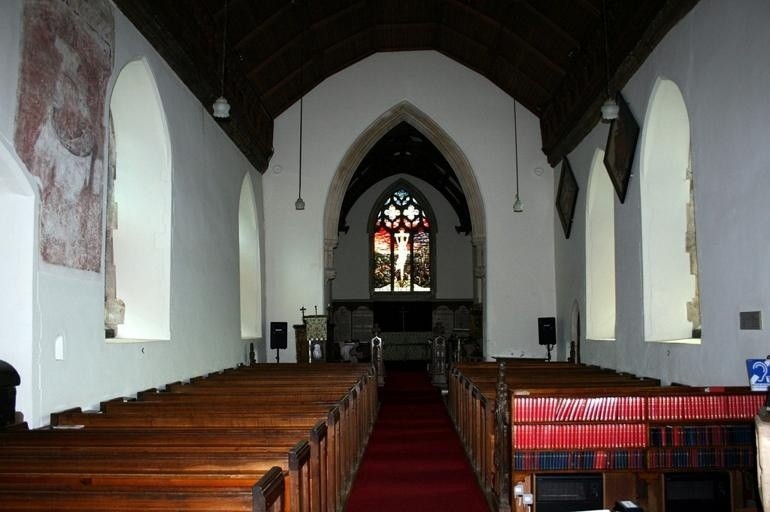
[
  {"xmin": 341, "ymin": 340, "xmax": 360, "ymax": 363},
  {"xmin": 427, "ymin": 345, "xmax": 432, "ymax": 371},
  {"xmin": 460, "ymin": 334, "xmax": 482, "ymax": 362}
]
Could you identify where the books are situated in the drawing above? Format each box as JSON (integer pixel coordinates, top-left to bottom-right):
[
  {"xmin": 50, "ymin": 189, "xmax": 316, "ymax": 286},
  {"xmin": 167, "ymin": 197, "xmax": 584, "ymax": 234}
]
[
  {"xmin": 705, "ymin": 387, "xmax": 726, "ymax": 393},
  {"xmin": 514, "ymin": 394, "xmax": 770, "ymax": 470}
]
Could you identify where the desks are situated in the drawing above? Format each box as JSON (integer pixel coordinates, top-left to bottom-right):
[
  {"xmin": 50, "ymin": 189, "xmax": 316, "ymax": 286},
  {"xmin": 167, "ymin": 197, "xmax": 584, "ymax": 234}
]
[{"xmin": 448, "ymin": 361, "xmax": 662, "ymax": 495}]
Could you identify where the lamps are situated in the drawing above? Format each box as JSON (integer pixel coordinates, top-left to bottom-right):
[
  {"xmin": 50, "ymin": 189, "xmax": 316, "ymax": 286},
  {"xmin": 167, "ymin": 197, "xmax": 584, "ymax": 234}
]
[
  {"xmin": 213, "ymin": 1, "xmax": 231, "ymax": 118},
  {"xmin": 513, "ymin": 66, "xmax": 525, "ymax": 212},
  {"xmin": 600, "ymin": 1, "xmax": 620, "ymax": 120},
  {"xmin": 294, "ymin": 50, "xmax": 305, "ymax": 210}
]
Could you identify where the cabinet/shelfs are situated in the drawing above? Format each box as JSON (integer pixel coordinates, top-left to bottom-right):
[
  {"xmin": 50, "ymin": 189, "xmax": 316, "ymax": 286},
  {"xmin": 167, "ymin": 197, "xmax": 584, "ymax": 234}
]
[{"xmin": 511, "ymin": 390, "xmax": 769, "ymax": 512}]
